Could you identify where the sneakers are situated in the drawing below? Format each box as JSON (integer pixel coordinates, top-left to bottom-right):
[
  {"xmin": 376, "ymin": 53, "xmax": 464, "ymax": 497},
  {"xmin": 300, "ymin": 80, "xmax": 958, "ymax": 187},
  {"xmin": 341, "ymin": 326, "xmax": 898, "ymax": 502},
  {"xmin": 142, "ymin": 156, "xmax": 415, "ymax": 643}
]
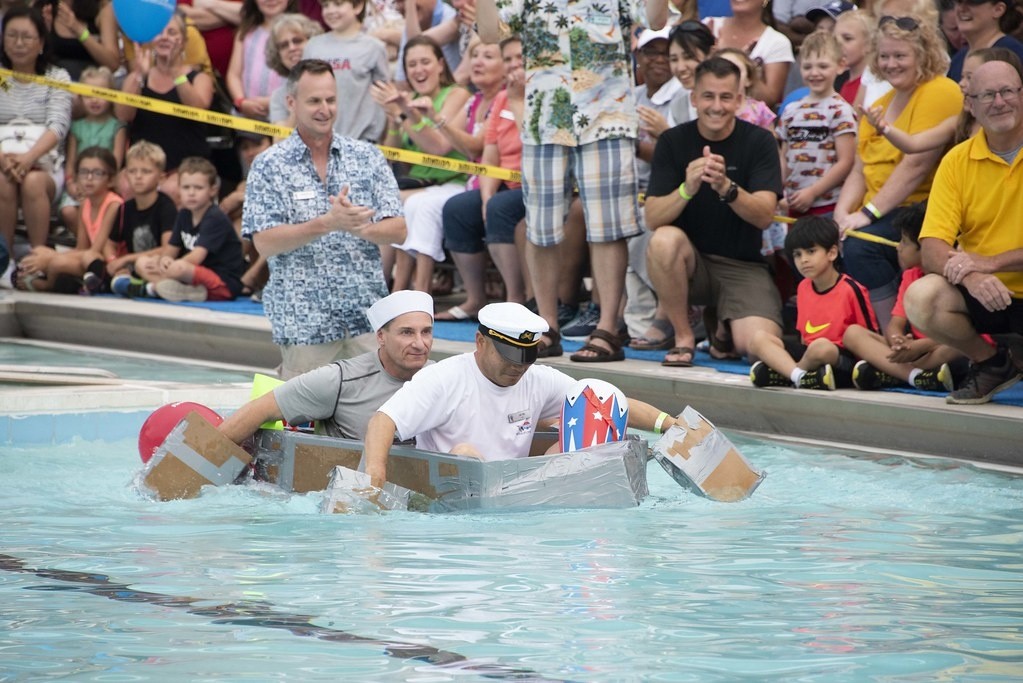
[
  {"xmin": 799, "ymin": 364, "xmax": 835, "ymax": 392},
  {"xmin": 914, "ymin": 362, "xmax": 953, "ymax": 393},
  {"xmin": 946, "ymin": 349, "xmax": 1021, "ymax": 404},
  {"xmin": 557, "ymin": 304, "xmax": 590, "ymax": 329},
  {"xmin": 749, "ymin": 360, "xmax": 794, "ymax": 387},
  {"xmin": 560, "ymin": 302, "xmax": 601, "ymax": 341},
  {"xmin": 852, "ymin": 360, "xmax": 916, "ymax": 391}
]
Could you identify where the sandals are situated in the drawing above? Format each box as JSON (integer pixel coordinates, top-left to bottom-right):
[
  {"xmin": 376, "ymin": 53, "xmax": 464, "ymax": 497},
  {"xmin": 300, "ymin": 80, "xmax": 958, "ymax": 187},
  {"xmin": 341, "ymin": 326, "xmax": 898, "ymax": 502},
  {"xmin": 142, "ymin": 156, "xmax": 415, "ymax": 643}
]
[
  {"xmin": 570, "ymin": 328, "xmax": 625, "ymax": 363},
  {"xmin": 627, "ymin": 319, "xmax": 684, "ymax": 348},
  {"xmin": 537, "ymin": 326, "xmax": 563, "ymax": 358},
  {"xmin": 435, "ymin": 306, "xmax": 479, "ymax": 324}
]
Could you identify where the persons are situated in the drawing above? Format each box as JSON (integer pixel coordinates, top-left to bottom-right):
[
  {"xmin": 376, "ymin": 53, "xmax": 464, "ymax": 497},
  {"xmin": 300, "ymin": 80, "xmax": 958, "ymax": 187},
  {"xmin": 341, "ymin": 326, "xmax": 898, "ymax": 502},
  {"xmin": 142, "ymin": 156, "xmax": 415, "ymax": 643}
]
[
  {"xmin": 241, "ymin": 59, "xmax": 407, "ymax": 382},
  {"xmin": 364, "ymin": 302, "xmax": 679, "ymax": 502},
  {"xmin": 215, "ymin": 290, "xmax": 434, "ymax": 444},
  {"xmin": 0, "ymin": 0, "xmax": 1023, "ymax": 406}
]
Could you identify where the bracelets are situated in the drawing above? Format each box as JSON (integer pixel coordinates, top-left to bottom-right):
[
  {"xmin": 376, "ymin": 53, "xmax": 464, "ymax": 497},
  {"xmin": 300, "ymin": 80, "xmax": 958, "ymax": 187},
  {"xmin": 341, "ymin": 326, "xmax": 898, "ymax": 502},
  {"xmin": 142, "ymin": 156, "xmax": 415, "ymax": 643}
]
[
  {"xmin": 654, "ymin": 412, "xmax": 670, "ymax": 434},
  {"xmin": 387, "ymin": 129, "xmax": 400, "ymax": 136},
  {"xmin": 679, "ymin": 182, "xmax": 692, "ymax": 200},
  {"xmin": 866, "ymin": 202, "xmax": 881, "ymax": 219},
  {"xmin": 173, "ymin": 74, "xmax": 188, "ymax": 86},
  {"xmin": 411, "ymin": 116, "xmax": 427, "ymax": 132},
  {"xmin": 79, "ymin": 29, "xmax": 89, "ymax": 42},
  {"xmin": 434, "ymin": 116, "xmax": 446, "ymax": 129},
  {"xmin": 861, "ymin": 207, "xmax": 878, "ymax": 222}
]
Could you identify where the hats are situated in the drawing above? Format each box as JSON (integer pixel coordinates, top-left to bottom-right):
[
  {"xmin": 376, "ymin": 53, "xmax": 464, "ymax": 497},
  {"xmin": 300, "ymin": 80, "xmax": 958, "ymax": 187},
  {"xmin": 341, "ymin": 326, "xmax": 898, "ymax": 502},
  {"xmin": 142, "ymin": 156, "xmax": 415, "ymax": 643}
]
[
  {"xmin": 637, "ymin": 25, "xmax": 672, "ymax": 50},
  {"xmin": 478, "ymin": 302, "xmax": 550, "ymax": 365},
  {"xmin": 366, "ymin": 289, "xmax": 434, "ymax": 335},
  {"xmin": 805, "ymin": 0, "xmax": 856, "ymax": 22}
]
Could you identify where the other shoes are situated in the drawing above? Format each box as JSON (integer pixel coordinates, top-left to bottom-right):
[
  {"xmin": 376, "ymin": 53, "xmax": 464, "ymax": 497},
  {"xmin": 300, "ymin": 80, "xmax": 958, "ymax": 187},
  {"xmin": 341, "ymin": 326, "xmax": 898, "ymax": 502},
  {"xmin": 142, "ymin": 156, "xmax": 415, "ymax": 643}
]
[
  {"xmin": 526, "ymin": 296, "xmax": 538, "ymax": 314},
  {"xmin": 0, "ymin": 259, "xmax": 264, "ymax": 304}
]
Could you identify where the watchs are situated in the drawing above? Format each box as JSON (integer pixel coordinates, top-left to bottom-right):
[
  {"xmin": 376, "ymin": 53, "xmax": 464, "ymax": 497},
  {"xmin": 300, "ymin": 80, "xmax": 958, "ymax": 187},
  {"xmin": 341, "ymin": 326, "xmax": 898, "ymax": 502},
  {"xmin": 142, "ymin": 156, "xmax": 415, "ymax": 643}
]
[{"xmin": 719, "ymin": 180, "xmax": 738, "ymax": 204}]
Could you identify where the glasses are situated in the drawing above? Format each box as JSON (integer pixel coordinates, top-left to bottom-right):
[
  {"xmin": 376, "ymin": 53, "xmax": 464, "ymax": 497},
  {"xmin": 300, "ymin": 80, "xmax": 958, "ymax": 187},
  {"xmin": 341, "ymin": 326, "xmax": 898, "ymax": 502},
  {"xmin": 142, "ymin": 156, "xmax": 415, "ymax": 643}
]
[
  {"xmin": 77, "ymin": 167, "xmax": 109, "ymax": 181},
  {"xmin": 642, "ymin": 46, "xmax": 669, "ymax": 61},
  {"xmin": 969, "ymin": 87, "xmax": 1021, "ymax": 103},
  {"xmin": 3, "ymin": 31, "xmax": 40, "ymax": 48},
  {"xmin": 276, "ymin": 38, "xmax": 304, "ymax": 49},
  {"xmin": 878, "ymin": 15, "xmax": 919, "ymax": 32}
]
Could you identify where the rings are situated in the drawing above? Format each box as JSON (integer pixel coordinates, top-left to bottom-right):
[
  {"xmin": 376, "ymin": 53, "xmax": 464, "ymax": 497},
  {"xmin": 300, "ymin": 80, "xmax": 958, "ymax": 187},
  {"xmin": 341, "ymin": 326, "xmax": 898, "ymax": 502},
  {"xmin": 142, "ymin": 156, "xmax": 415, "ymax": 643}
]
[{"xmin": 958, "ymin": 263, "xmax": 962, "ymax": 268}]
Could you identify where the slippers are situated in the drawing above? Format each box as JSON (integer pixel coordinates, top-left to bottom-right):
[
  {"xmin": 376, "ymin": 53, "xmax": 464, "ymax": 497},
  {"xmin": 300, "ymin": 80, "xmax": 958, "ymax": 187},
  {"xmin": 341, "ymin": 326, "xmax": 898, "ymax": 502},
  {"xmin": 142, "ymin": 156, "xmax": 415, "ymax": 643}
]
[
  {"xmin": 704, "ymin": 305, "xmax": 742, "ymax": 361},
  {"xmin": 661, "ymin": 347, "xmax": 694, "ymax": 366}
]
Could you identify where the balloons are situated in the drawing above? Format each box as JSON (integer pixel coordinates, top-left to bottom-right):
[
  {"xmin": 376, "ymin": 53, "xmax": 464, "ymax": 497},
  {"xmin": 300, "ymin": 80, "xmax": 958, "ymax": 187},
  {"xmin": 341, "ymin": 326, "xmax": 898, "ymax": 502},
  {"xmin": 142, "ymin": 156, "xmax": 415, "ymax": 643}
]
[{"xmin": 112, "ymin": 1, "xmax": 176, "ymax": 45}]
